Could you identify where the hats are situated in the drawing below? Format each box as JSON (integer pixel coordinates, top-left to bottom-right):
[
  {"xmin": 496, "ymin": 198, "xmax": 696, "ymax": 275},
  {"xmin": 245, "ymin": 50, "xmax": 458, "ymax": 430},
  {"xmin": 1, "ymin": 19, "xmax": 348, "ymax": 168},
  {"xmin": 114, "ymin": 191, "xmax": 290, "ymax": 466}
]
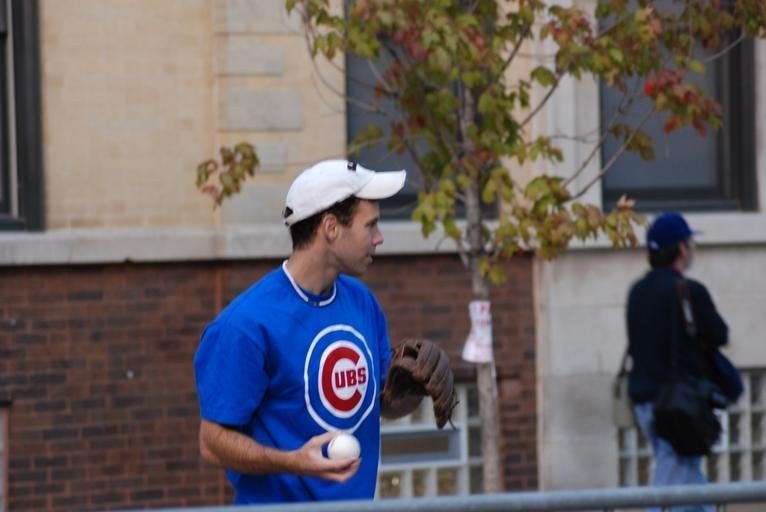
[
  {"xmin": 646, "ymin": 213, "xmax": 701, "ymax": 248},
  {"xmin": 281, "ymin": 159, "xmax": 408, "ymax": 227}
]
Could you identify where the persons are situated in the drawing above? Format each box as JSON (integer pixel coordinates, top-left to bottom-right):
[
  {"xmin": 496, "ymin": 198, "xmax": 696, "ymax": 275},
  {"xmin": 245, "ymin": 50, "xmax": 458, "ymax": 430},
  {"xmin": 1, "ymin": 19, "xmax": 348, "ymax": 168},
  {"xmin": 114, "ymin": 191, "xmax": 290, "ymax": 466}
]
[
  {"xmin": 191, "ymin": 158, "xmax": 457, "ymax": 508},
  {"xmin": 627, "ymin": 213, "xmax": 729, "ymax": 512}
]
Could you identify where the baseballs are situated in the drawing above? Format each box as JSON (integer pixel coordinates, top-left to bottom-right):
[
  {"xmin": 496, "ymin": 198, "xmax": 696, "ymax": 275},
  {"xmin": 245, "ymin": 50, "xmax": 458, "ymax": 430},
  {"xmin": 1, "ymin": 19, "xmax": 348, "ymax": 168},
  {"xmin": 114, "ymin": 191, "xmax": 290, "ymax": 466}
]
[{"xmin": 328, "ymin": 434, "xmax": 360, "ymax": 464}]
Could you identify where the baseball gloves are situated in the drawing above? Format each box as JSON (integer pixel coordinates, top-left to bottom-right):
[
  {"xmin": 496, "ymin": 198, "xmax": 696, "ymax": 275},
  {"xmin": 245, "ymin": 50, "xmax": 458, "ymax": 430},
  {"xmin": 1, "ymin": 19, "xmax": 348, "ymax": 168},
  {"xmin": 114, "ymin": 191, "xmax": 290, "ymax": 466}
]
[{"xmin": 384, "ymin": 338, "xmax": 458, "ymax": 431}]
